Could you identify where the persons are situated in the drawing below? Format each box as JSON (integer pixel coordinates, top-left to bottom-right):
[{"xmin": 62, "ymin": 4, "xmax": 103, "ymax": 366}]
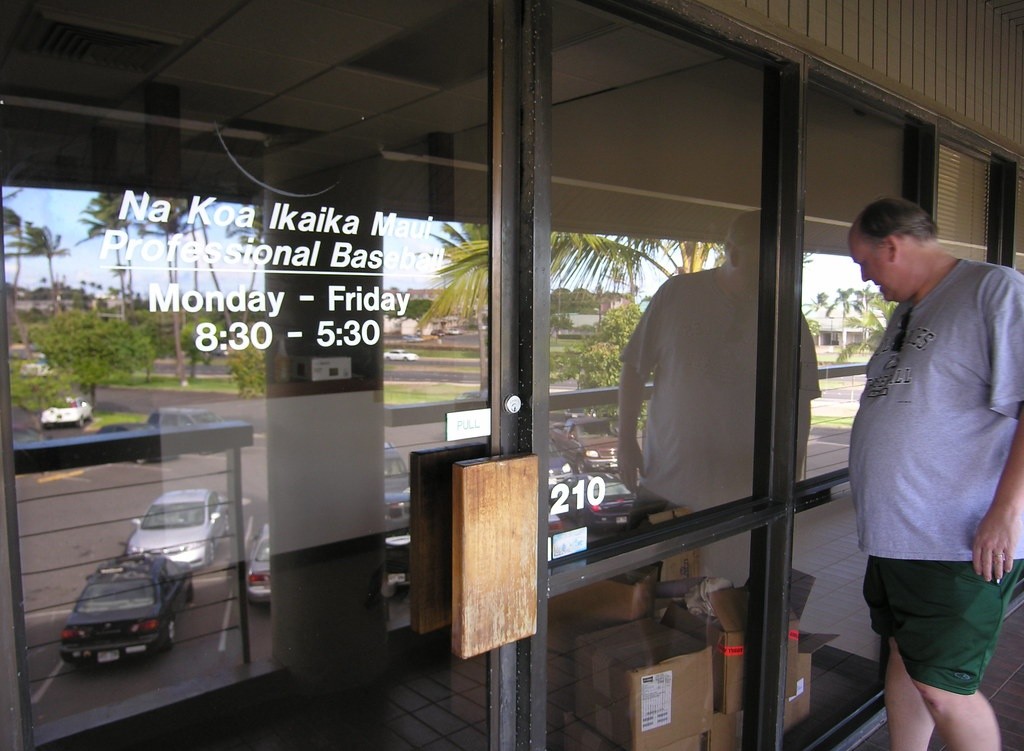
[
  {"xmin": 614, "ymin": 208, "xmax": 820, "ymax": 581},
  {"xmin": 849, "ymin": 198, "xmax": 1024, "ymax": 751}
]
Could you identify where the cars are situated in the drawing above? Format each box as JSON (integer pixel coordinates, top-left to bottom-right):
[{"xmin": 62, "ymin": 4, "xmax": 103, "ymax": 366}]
[
  {"xmin": 383, "ymin": 349, "xmax": 420, "ymax": 362},
  {"xmin": 125, "ymin": 488, "xmax": 226, "ymax": 569},
  {"xmin": 41, "ymin": 396, "xmax": 94, "ymax": 430},
  {"xmin": 59, "ymin": 549, "xmax": 194, "ymax": 667},
  {"xmin": 246, "ymin": 522, "xmax": 271, "ymax": 609},
  {"xmin": 375, "ymin": 413, "xmax": 638, "ymax": 588}
]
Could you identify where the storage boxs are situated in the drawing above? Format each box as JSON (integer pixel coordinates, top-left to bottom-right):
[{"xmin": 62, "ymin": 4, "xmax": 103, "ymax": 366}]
[
  {"xmin": 709, "ymin": 633, "xmax": 840, "ymax": 751},
  {"xmin": 661, "ymin": 568, "xmax": 817, "ymax": 715},
  {"xmin": 644, "ymin": 504, "xmax": 701, "ymax": 582},
  {"xmin": 576, "ymin": 616, "xmax": 712, "ymax": 751},
  {"xmin": 563, "ymin": 711, "xmax": 703, "ymax": 751},
  {"xmin": 575, "ymin": 567, "xmax": 659, "ymax": 621}
]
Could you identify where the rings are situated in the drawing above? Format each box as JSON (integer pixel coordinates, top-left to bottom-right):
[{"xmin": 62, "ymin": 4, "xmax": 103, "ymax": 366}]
[{"xmin": 993, "ymin": 553, "xmax": 1003, "ymax": 558}]
